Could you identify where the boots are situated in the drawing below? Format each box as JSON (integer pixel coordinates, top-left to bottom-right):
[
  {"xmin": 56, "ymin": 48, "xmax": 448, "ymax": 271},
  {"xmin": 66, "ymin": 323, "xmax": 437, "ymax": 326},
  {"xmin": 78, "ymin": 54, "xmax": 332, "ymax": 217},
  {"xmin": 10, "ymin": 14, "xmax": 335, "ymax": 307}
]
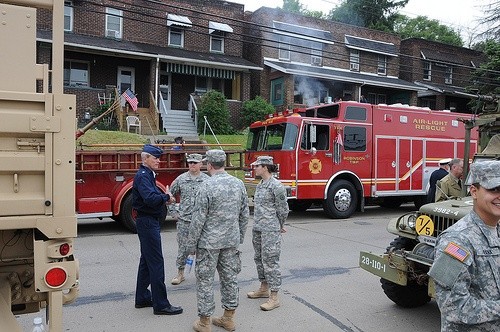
[
  {"xmin": 212, "ymin": 309, "xmax": 235, "ymax": 331},
  {"xmin": 193, "ymin": 315, "xmax": 212, "ymax": 332},
  {"xmin": 248, "ymin": 281, "xmax": 270, "ymax": 298},
  {"xmin": 171, "ymin": 268, "xmax": 185, "ymax": 284},
  {"xmin": 260, "ymin": 292, "xmax": 280, "ymax": 311}
]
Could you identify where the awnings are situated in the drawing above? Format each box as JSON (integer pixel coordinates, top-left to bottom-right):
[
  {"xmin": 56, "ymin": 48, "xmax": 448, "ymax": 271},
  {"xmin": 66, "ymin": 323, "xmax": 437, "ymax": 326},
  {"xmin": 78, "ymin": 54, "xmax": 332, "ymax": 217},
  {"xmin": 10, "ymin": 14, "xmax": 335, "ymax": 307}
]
[
  {"xmin": 208, "ymin": 20, "xmax": 233, "ymax": 34},
  {"xmin": 471, "ymin": 58, "xmax": 491, "ymax": 68},
  {"xmin": 272, "ymin": 20, "xmax": 336, "ymax": 46},
  {"xmin": 166, "ymin": 13, "xmax": 193, "ymax": 28},
  {"xmin": 344, "ymin": 34, "xmax": 400, "ymax": 57},
  {"xmin": 421, "ymin": 48, "xmax": 464, "ymax": 66}
]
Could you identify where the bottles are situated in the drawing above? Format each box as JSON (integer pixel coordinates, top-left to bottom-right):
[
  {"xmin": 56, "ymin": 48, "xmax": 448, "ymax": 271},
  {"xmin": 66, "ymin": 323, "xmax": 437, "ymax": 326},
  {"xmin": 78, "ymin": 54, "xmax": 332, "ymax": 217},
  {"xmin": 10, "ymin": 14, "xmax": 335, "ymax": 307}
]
[
  {"xmin": 29, "ymin": 317, "xmax": 47, "ymax": 332},
  {"xmin": 184, "ymin": 254, "xmax": 194, "ymax": 275}
]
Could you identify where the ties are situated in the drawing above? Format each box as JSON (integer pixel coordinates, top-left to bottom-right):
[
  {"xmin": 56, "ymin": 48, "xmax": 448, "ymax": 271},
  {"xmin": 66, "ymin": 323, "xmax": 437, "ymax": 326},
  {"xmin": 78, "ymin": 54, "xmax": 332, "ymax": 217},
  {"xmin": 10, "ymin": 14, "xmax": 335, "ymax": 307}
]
[{"xmin": 458, "ymin": 180, "xmax": 462, "ymax": 189}]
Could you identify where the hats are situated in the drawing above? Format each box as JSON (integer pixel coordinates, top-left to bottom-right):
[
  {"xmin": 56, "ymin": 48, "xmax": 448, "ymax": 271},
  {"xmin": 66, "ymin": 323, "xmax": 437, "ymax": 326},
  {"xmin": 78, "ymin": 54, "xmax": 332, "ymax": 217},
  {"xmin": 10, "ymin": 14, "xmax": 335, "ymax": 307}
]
[
  {"xmin": 440, "ymin": 158, "xmax": 452, "ymax": 165},
  {"xmin": 187, "ymin": 154, "xmax": 202, "ymax": 162},
  {"xmin": 470, "ymin": 161, "xmax": 500, "ymax": 189},
  {"xmin": 250, "ymin": 156, "xmax": 273, "ymax": 165},
  {"xmin": 142, "ymin": 144, "xmax": 163, "ymax": 158},
  {"xmin": 201, "ymin": 149, "xmax": 226, "ymax": 162}
]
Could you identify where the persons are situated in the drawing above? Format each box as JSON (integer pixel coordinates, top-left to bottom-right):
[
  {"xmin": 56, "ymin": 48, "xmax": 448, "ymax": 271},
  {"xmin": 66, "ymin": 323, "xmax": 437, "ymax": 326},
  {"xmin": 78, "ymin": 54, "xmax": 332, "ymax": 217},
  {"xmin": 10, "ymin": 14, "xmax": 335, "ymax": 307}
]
[
  {"xmin": 434, "ymin": 158, "xmax": 466, "ymax": 202},
  {"xmin": 169, "ymin": 153, "xmax": 211, "ymax": 285},
  {"xmin": 186, "ymin": 149, "xmax": 250, "ymax": 332},
  {"xmin": 132, "ymin": 144, "xmax": 183, "ymax": 315},
  {"xmin": 426, "ymin": 158, "xmax": 453, "ymax": 203},
  {"xmin": 247, "ymin": 156, "xmax": 289, "ymax": 311},
  {"xmin": 426, "ymin": 160, "xmax": 500, "ymax": 332}
]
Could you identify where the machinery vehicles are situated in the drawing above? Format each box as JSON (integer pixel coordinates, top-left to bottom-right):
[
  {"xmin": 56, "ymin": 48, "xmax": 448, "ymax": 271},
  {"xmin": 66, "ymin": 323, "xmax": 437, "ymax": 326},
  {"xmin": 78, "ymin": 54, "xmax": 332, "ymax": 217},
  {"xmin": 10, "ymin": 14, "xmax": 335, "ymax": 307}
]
[
  {"xmin": 0, "ymin": 13, "xmax": 81, "ymax": 331},
  {"xmin": 358, "ymin": 133, "xmax": 500, "ymax": 307},
  {"xmin": 76, "ymin": 143, "xmax": 212, "ymax": 233}
]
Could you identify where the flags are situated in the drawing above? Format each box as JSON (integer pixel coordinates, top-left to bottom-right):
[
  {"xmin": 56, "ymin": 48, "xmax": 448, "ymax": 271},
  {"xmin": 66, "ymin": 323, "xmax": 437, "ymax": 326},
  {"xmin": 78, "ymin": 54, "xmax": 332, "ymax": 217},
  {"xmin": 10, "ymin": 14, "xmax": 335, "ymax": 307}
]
[
  {"xmin": 337, "ymin": 133, "xmax": 343, "ymax": 146},
  {"xmin": 122, "ymin": 88, "xmax": 139, "ymax": 111}
]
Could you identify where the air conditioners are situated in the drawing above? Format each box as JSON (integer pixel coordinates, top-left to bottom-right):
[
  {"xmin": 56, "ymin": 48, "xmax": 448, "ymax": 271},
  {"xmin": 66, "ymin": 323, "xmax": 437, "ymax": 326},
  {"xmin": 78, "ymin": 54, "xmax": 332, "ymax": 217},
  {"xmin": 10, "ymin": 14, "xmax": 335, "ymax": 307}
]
[
  {"xmin": 314, "ymin": 57, "xmax": 322, "ymax": 63},
  {"xmin": 108, "ymin": 31, "xmax": 117, "ymax": 38},
  {"xmin": 350, "ymin": 64, "xmax": 356, "ymax": 70}
]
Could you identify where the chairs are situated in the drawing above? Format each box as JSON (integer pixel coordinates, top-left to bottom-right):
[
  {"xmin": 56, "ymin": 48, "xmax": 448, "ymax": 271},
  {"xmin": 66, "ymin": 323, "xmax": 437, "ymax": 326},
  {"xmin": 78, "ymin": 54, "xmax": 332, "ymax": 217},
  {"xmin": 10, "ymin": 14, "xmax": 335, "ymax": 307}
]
[
  {"xmin": 126, "ymin": 116, "xmax": 141, "ymax": 135},
  {"xmin": 317, "ymin": 133, "xmax": 328, "ymax": 150}
]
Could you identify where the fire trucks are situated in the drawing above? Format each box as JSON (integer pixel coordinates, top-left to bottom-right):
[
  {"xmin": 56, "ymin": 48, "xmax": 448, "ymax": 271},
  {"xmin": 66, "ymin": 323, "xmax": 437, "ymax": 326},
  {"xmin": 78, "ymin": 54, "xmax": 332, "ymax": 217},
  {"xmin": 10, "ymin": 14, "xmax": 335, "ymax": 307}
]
[{"xmin": 244, "ymin": 101, "xmax": 479, "ymax": 218}]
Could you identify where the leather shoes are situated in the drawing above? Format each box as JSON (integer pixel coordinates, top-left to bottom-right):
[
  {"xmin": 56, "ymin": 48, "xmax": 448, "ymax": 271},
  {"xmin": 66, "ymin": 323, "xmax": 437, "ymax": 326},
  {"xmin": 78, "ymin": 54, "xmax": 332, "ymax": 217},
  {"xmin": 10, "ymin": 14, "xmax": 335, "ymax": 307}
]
[
  {"xmin": 154, "ymin": 304, "xmax": 183, "ymax": 314},
  {"xmin": 135, "ymin": 300, "xmax": 153, "ymax": 308}
]
